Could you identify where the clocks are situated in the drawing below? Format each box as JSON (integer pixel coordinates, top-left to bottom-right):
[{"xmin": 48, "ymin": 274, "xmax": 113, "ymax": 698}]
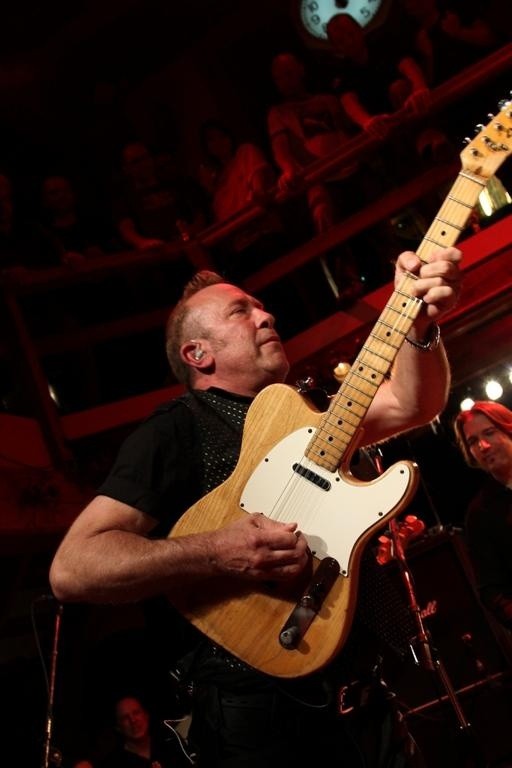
[{"xmin": 291, "ymin": 2, "xmax": 386, "ymax": 45}]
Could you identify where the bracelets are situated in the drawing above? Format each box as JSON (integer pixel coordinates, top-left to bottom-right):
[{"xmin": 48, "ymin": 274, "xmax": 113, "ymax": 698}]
[{"xmin": 404, "ymin": 321, "xmax": 440, "ymax": 352}]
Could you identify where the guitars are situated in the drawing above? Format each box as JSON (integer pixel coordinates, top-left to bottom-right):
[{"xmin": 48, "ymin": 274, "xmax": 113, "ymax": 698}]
[{"xmin": 163, "ymin": 82, "xmax": 512, "ymax": 680}]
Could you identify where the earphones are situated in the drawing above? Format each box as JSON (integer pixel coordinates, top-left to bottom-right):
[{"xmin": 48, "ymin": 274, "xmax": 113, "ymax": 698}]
[{"xmin": 194, "ymin": 351, "xmax": 205, "ymax": 359}]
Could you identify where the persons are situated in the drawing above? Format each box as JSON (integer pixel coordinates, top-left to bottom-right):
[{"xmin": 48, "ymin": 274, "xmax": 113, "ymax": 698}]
[
  {"xmin": 0, "ymin": 2, "xmax": 510, "ymax": 271},
  {"xmin": 109, "ymin": 691, "xmax": 164, "ymax": 767},
  {"xmin": 72, "ymin": 757, "xmax": 94, "ymax": 768},
  {"xmin": 48, "ymin": 245, "xmax": 463, "ymax": 768},
  {"xmin": 455, "ymin": 400, "xmax": 512, "ymax": 627}
]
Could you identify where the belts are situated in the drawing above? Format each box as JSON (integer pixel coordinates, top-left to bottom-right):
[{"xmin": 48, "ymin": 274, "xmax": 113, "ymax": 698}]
[{"xmin": 207, "ymin": 648, "xmax": 385, "ymax": 715}]
